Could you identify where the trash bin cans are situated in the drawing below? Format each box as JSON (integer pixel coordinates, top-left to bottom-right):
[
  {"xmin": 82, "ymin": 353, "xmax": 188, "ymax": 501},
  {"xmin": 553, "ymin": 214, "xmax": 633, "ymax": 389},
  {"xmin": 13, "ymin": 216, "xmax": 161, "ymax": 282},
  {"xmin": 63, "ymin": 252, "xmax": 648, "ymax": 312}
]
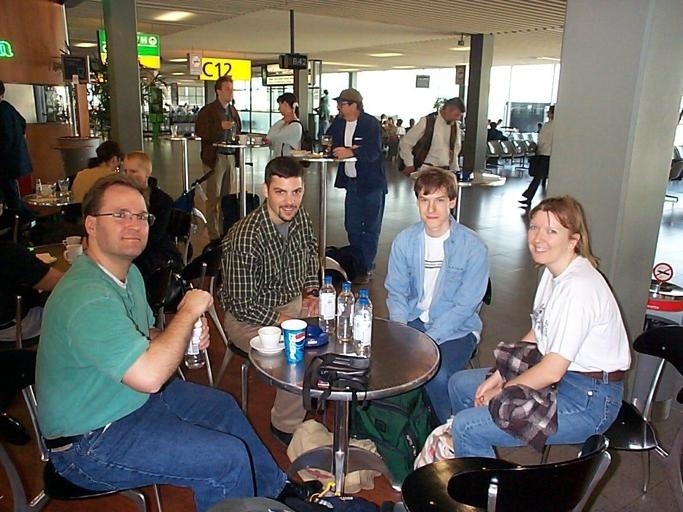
[{"xmin": 632, "ymin": 314, "xmax": 680, "ymax": 422}]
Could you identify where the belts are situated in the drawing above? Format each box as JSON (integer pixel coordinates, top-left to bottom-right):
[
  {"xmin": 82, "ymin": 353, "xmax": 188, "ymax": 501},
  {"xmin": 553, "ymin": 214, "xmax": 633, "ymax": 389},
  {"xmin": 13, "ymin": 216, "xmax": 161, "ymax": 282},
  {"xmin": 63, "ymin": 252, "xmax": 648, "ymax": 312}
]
[
  {"xmin": 1, "ymin": 309, "xmax": 29, "ymax": 331},
  {"xmin": 574, "ymin": 370, "xmax": 626, "ymax": 382}
]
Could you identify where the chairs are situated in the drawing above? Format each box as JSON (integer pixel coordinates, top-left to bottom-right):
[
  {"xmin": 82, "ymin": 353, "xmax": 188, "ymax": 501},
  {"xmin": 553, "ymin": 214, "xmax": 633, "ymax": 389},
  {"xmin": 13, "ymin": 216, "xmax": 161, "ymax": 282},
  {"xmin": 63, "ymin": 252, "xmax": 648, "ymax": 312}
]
[
  {"xmin": 16, "ymin": 291, "xmax": 164, "ymax": 512},
  {"xmin": 470, "ymin": 276, "xmax": 491, "ymax": 369},
  {"xmin": 400, "ymin": 431, "xmax": 606, "ymax": 507},
  {"xmin": 598, "ymin": 325, "xmax": 682, "ymax": 492},
  {"xmin": 200, "ymin": 251, "xmax": 352, "ymax": 414},
  {"xmin": 0, "ymin": 175, "xmax": 203, "ymax": 351},
  {"xmin": 377, "ymin": 123, "xmax": 536, "ymax": 171}
]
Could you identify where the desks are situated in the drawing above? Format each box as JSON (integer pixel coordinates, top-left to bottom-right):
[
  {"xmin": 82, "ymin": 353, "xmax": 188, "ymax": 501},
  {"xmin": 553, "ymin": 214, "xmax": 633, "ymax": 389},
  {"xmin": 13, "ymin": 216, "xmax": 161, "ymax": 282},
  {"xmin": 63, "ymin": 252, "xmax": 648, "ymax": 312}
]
[
  {"xmin": 245, "ymin": 314, "xmax": 442, "ymax": 509},
  {"xmin": 212, "ymin": 141, "xmax": 268, "ymax": 220},
  {"xmin": 301, "ymin": 153, "xmax": 359, "ymax": 285},
  {"xmin": 164, "ymin": 135, "xmax": 202, "ymax": 193}
]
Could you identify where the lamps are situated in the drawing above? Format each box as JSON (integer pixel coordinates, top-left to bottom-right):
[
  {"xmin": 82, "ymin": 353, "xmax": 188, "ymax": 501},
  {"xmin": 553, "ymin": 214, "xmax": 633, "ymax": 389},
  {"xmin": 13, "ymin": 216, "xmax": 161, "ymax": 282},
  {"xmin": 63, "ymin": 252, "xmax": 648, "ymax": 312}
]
[{"xmin": 458, "ymin": 33, "xmax": 467, "ymax": 47}]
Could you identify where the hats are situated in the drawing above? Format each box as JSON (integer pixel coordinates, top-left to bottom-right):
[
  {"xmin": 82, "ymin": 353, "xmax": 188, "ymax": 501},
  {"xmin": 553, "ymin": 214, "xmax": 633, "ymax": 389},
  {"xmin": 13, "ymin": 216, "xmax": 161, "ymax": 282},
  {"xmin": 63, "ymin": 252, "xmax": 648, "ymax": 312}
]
[{"xmin": 331, "ymin": 89, "xmax": 363, "ymax": 104}]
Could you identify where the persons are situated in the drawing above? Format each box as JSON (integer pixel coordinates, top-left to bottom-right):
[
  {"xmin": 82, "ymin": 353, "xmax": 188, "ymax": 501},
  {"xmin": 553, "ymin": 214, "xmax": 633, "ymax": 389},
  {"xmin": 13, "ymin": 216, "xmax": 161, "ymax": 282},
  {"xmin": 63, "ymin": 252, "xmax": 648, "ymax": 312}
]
[
  {"xmin": 195, "ymin": 77, "xmax": 240, "ymax": 243},
  {"xmin": 1, "ymin": 81, "xmax": 173, "ymax": 341},
  {"xmin": 449, "ymin": 194, "xmax": 633, "ymax": 459},
  {"xmin": 164, "ymin": 101, "xmax": 200, "ymax": 116},
  {"xmin": 218, "ymin": 157, "xmax": 333, "ymax": 448},
  {"xmin": 384, "ymin": 168, "xmax": 490, "ymax": 425},
  {"xmin": 35, "ymin": 170, "xmax": 323, "ymax": 512},
  {"xmin": 324, "ymin": 88, "xmax": 386, "ymax": 284},
  {"xmin": 315, "ymin": 90, "xmax": 329, "ymax": 140},
  {"xmin": 519, "ymin": 105, "xmax": 555, "ymax": 205},
  {"xmin": 536, "ymin": 123, "xmax": 542, "ymax": 134},
  {"xmin": 376, "ymin": 114, "xmax": 414, "ymax": 161},
  {"xmin": 487, "ymin": 118, "xmax": 509, "ymax": 141},
  {"xmin": 264, "ymin": 93, "xmax": 303, "ymax": 159},
  {"xmin": 398, "ymin": 97, "xmax": 466, "ymax": 216},
  {"xmin": 329, "ymin": 115, "xmax": 335, "ymax": 123}
]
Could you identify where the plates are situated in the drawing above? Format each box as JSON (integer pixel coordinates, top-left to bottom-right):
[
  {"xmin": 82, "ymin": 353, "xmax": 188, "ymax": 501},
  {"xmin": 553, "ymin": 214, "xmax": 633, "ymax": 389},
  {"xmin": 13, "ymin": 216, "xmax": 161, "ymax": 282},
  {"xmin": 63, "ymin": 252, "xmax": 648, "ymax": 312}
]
[{"xmin": 249, "ymin": 335, "xmax": 285, "ymax": 354}]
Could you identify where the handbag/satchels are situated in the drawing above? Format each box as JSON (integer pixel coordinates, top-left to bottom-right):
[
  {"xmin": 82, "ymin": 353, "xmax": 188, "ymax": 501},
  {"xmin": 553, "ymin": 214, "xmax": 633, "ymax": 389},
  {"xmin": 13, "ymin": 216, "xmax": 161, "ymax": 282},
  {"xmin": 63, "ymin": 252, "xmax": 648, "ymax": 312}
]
[
  {"xmin": 319, "ymin": 245, "xmax": 364, "ymax": 287},
  {"xmin": 529, "ymin": 155, "xmax": 550, "ymax": 177},
  {"xmin": 286, "ymin": 387, "xmax": 455, "ymax": 494}
]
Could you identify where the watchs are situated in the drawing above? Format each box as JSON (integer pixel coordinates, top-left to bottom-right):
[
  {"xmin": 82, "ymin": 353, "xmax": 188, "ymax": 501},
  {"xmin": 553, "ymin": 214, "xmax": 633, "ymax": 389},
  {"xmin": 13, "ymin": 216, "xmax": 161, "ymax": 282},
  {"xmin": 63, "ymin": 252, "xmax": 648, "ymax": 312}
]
[{"xmin": 307, "ymin": 289, "xmax": 320, "ymax": 298}]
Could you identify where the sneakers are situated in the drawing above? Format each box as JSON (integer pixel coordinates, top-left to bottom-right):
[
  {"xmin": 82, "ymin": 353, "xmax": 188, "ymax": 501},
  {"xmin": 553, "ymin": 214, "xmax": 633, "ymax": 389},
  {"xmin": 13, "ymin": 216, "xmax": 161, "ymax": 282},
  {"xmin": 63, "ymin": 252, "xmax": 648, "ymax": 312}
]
[
  {"xmin": 518, "ymin": 201, "xmax": 531, "ymax": 205},
  {"xmin": 350, "ymin": 268, "xmax": 375, "ymax": 284},
  {"xmin": 269, "ymin": 421, "xmax": 294, "ymax": 447},
  {"xmin": 267, "ymin": 480, "xmax": 323, "ymax": 506}
]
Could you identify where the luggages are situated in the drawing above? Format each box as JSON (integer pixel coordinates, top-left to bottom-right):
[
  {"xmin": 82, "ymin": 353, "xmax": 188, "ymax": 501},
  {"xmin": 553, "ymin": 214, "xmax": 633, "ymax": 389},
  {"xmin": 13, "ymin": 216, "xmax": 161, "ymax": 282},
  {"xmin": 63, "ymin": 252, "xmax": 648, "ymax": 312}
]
[{"xmin": 221, "ymin": 162, "xmax": 260, "ymax": 236}]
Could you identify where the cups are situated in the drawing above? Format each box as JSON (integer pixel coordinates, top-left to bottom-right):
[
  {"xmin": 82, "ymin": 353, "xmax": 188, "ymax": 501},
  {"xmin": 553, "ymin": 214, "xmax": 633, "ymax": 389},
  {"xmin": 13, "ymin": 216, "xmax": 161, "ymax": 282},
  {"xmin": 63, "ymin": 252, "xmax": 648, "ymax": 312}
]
[
  {"xmin": 322, "ymin": 135, "xmax": 332, "ymax": 156},
  {"xmin": 280, "ymin": 319, "xmax": 308, "ymax": 364},
  {"xmin": 58, "ymin": 179, "xmax": 70, "ymax": 196},
  {"xmin": 259, "ymin": 326, "xmax": 281, "ymax": 348},
  {"xmin": 62, "ymin": 235, "xmax": 82, "ymax": 265},
  {"xmin": 170, "ymin": 125, "xmax": 178, "ymax": 138},
  {"xmin": 239, "ymin": 135, "xmax": 248, "ymax": 145},
  {"xmin": 228, "ymin": 120, "xmax": 236, "ymax": 142},
  {"xmin": 47, "ymin": 182, "xmax": 57, "ymax": 199}
]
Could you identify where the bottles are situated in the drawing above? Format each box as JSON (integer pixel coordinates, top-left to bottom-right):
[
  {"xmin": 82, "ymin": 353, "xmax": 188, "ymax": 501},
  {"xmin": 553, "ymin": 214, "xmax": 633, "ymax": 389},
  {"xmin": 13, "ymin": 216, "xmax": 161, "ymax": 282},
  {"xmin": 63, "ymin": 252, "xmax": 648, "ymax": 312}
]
[
  {"xmin": 318, "ymin": 276, "xmax": 373, "ymax": 358},
  {"xmin": 184, "ymin": 317, "xmax": 206, "ymax": 371}
]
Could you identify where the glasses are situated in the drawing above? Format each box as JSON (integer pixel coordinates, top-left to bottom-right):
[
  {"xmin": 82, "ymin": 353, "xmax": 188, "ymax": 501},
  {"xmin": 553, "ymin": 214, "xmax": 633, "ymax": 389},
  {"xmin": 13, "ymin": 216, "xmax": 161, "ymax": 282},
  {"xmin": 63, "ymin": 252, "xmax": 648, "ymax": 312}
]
[{"xmin": 85, "ymin": 209, "xmax": 156, "ymax": 228}]
